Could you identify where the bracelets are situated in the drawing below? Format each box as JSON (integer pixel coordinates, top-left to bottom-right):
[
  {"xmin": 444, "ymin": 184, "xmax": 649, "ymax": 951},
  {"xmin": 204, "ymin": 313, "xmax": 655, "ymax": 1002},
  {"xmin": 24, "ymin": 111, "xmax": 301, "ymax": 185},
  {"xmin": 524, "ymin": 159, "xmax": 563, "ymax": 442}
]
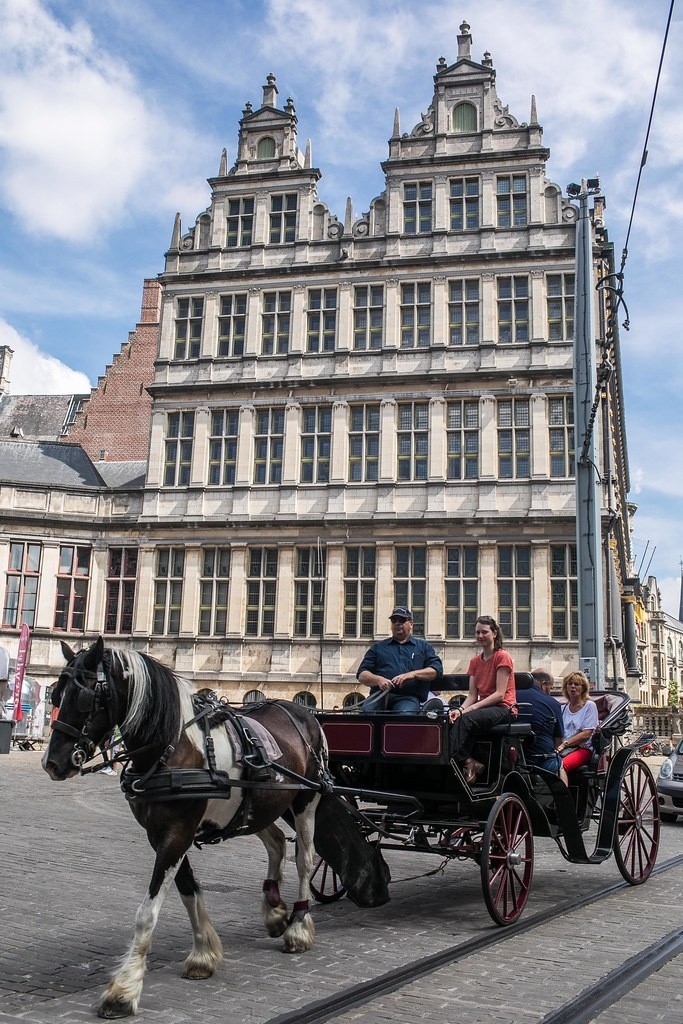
[
  {"xmin": 458, "ymin": 709, "xmax": 462, "ymax": 715},
  {"xmin": 461, "ymin": 707, "xmax": 464, "ymax": 710},
  {"xmin": 562, "ymin": 743, "xmax": 566, "ymax": 748}
]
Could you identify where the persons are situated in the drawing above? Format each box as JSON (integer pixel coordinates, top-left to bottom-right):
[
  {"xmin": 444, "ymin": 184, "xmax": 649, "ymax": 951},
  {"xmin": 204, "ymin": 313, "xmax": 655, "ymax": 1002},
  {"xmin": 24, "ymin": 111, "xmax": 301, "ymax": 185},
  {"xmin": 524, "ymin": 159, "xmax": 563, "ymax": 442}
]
[
  {"xmin": 449, "ymin": 616, "xmax": 518, "ymax": 786},
  {"xmin": 546, "ymin": 671, "xmax": 599, "ymax": 809},
  {"xmin": 420, "ymin": 690, "xmax": 451, "ymax": 722},
  {"xmin": 100, "ymin": 724, "xmax": 124, "ymax": 775},
  {"xmin": 515, "ymin": 667, "xmax": 569, "ymax": 787},
  {"xmin": 333, "ymin": 705, "xmax": 339, "ymax": 714},
  {"xmin": 356, "ymin": 607, "xmax": 443, "ymax": 714}
]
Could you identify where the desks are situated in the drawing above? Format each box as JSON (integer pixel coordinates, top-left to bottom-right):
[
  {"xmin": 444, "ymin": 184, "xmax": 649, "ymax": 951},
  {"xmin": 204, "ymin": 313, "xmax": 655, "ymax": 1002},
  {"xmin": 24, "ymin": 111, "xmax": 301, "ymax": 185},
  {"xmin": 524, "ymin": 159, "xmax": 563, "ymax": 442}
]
[{"xmin": 12, "ymin": 739, "xmax": 44, "ymax": 751}]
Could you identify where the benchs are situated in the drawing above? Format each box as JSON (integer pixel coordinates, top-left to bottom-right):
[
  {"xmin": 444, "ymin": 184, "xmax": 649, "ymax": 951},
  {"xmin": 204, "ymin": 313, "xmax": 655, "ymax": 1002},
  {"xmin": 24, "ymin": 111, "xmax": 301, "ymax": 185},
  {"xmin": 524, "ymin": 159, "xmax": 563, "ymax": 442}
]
[
  {"xmin": 550, "ymin": 691, "xmax": 631, "ymax": 777},
  {"xmin": 430, "ymin": 674, "xmax": 534, "ymax": 735}
]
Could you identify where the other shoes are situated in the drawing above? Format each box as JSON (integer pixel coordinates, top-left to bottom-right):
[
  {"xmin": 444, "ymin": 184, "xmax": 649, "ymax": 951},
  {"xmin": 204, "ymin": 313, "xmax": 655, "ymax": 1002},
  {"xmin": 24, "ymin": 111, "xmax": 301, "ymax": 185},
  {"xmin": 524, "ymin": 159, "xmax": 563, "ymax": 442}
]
[{"xmin": 464, "ymin": 757, "xmax": 485, "ymax": 787}]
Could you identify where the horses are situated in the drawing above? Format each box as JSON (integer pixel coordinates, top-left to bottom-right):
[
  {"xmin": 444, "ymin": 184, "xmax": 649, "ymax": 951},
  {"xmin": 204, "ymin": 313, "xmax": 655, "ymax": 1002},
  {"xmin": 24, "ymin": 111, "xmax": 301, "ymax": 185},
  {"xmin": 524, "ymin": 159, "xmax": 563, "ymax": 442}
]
[{"xmin": 40, "ymin": 635, "xmax": 329, "ymax": 1020}]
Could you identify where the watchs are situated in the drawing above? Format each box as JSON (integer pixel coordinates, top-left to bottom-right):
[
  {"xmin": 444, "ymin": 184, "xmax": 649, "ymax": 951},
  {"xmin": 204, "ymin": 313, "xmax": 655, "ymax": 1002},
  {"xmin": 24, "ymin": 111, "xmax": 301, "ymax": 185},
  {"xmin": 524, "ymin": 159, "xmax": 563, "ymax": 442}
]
[{"xmin": 564, "ymin": 741, "xmax": 569, "ymax": 746}]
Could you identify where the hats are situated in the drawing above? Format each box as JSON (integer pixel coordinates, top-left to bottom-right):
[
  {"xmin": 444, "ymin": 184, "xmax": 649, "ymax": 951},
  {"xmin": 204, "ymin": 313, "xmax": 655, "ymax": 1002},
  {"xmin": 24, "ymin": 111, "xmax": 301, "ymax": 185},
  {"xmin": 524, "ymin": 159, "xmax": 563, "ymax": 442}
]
[{"xmin": 388, "ymin": 608, "xmax": 412, "ymax": 620}]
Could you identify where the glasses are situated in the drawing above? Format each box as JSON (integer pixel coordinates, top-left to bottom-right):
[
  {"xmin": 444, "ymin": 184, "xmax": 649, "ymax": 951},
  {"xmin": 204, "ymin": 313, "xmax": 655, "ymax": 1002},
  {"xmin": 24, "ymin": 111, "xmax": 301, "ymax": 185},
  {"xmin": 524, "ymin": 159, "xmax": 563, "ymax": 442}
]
[{"xmin": 391, "ymin": 618, "xmax": 411, "ymax": 623}]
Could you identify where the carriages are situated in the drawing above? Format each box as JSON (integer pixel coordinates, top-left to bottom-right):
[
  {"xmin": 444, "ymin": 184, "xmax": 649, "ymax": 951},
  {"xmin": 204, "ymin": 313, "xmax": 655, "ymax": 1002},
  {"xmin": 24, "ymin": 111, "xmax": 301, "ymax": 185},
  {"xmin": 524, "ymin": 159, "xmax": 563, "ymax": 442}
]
[{"xmin": 40, "ymin": 632, "xmax": 662, "ymax": 1019}]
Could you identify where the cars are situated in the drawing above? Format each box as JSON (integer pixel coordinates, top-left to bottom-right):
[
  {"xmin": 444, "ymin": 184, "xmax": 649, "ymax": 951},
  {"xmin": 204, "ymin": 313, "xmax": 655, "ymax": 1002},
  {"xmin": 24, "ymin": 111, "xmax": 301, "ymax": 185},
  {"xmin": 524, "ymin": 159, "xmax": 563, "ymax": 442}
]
[{"xmin": 655, "ymin": 737, "xmax": 683, "ymax": 823}]
